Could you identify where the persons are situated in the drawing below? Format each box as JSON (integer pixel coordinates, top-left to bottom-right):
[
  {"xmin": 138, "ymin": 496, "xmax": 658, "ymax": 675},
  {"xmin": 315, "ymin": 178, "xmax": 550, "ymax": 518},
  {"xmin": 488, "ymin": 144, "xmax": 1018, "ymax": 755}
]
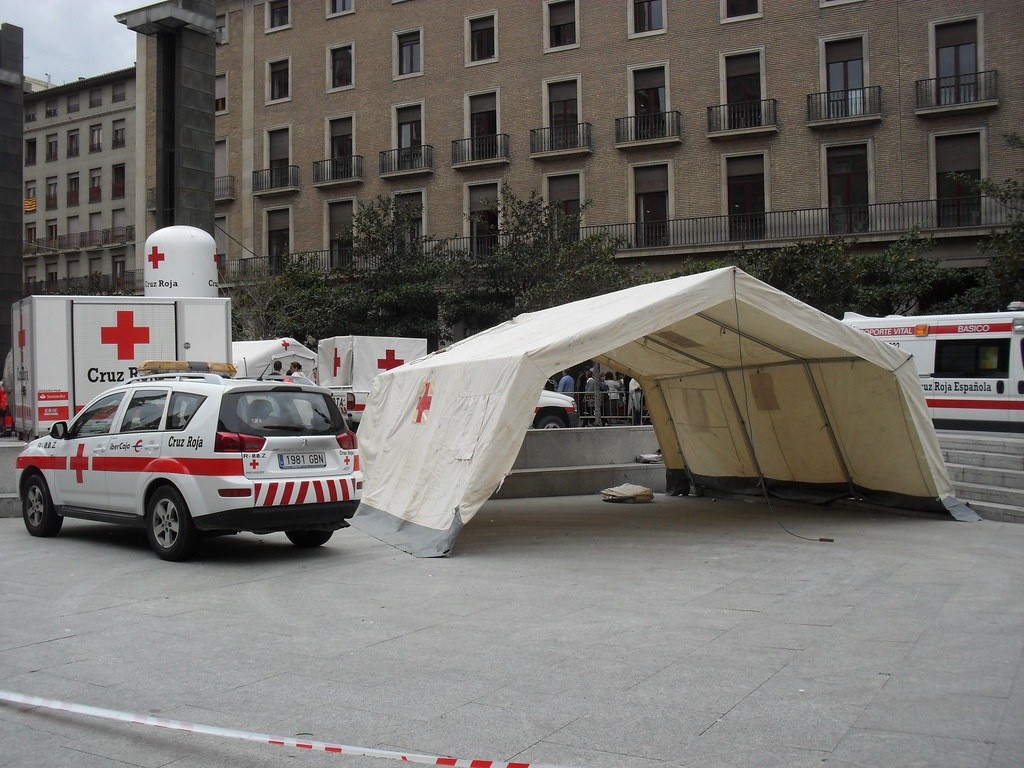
[
  {"xmin": 268, "ymin": 361, "xmax": 319, "ymax": 385},
  {"xmin": 0, "ymin": 381, "xmax": 13, "ymax": 438},
  {"xmin": 545, "ymin": 366, "xmax": 651, "ymax": 427}
]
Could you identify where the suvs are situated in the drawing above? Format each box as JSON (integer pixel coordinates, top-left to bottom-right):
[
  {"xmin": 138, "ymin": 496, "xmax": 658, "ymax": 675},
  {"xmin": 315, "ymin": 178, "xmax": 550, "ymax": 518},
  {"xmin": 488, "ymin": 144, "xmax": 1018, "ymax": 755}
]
[{"xmin": 531, "ymin": 388, "xmax": 580, "ymax": 430}]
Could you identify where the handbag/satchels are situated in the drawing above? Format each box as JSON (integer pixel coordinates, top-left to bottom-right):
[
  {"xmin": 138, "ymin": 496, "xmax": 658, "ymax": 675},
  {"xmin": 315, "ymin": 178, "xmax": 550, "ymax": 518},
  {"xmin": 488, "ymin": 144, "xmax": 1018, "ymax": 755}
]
[{"xmin": 600, "ymin": 387, "xmax": 609, "ymax": 402}]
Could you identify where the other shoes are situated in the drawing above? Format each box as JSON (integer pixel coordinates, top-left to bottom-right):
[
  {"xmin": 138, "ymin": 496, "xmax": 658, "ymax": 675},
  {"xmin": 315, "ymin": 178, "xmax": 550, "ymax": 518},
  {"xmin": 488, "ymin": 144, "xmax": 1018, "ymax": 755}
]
[{"xmin": 582, "ymin": 419, "xmax": 609, "ymax": 426}]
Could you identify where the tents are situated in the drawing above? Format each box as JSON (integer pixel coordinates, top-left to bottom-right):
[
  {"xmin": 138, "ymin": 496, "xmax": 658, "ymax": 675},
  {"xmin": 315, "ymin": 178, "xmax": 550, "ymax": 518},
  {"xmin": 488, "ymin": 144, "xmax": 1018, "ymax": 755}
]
[
  {"xmin": 344, "ymin": 266, "xmax": 983, "ymax": 558},
  {"xmin": 231, "ymin": 337, "xmax": 318, "ymax": 381},
  {"xmin": 318, "ymin": 336, "xmax": 427, "ymax": 392}
]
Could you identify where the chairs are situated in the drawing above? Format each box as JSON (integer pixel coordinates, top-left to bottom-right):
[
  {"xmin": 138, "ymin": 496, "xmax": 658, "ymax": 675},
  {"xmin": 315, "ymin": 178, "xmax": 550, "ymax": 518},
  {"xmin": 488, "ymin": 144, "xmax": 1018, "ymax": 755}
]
[
  {"xmin": 134, "ymin": 406, "xmax": 163, "ymax": 429},
  {"xmin": 248, "ymin": 400, "xmax": 273, "ymax": 428},
  {"xmin": 178, "ymin": 399, "xmax": 200, "ymax": 428}
]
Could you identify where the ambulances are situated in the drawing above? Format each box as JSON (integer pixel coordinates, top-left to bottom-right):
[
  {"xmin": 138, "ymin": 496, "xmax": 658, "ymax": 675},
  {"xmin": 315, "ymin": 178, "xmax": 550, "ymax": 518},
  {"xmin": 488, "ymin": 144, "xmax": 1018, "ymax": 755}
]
[
  {"xmin": 8, "ymin": 224, "xmax": 427, "ymax": 446},
  {"xmin": 13, "ymin": 361, "xmax": 367, "ymax": 561},
  {"xmin": 839, "ymin": 301, "xmax": 1024, "ymax": 432}
]
